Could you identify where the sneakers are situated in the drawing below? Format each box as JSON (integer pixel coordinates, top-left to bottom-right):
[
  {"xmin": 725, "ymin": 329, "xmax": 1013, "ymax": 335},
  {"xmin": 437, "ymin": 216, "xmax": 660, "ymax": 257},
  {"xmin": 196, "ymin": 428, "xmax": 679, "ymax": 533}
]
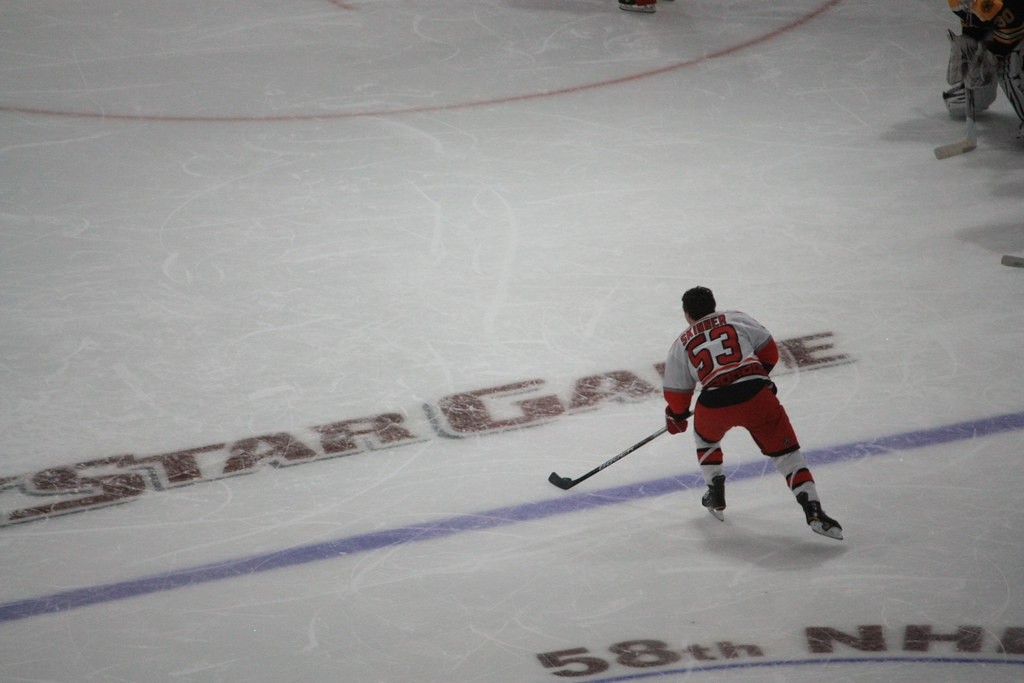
[
  {"xmin": 702, "ymin": 475, "xmax": 726, "ymax": 522},
  {"xmin": 797, "ymin": 492, "xmax": 843, "ymax": 540},
  {"xmin": 618, "ymin": 0, "xmax": 657, "ymax": 13}
]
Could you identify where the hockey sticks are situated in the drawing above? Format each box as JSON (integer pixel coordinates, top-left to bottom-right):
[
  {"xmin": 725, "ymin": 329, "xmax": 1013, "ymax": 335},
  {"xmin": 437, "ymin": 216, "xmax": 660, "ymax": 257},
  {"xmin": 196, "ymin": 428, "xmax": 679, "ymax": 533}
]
[
  {"xmin": 932, "ymin": 59, "xmax": 978, "ymax": 160},
  {"xmin": 547, "ymin": 409, "xmax": 694, "ymax": 491},
  {"xmin": 1001, "ymin": 254, "xmax": 1024, "ymax": 268}
]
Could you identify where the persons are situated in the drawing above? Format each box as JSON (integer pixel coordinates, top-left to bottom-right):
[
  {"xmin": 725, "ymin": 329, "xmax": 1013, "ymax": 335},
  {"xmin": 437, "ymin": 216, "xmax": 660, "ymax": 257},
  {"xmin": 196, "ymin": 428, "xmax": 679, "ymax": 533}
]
[
  {"xmin": 942, "ymin": 0, "xmax": 1024, "ymax": 141},
  {"xmin": 664, "ymin": 285, "xmax": 844, "ymax": 543}
]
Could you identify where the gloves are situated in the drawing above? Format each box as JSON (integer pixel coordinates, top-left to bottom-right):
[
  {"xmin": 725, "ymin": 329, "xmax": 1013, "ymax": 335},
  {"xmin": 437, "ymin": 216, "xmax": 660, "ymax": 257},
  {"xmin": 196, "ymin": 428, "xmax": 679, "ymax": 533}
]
[{"xmin": 665, "ymin": 405, "xmax": 687, "ymax": 434}]
